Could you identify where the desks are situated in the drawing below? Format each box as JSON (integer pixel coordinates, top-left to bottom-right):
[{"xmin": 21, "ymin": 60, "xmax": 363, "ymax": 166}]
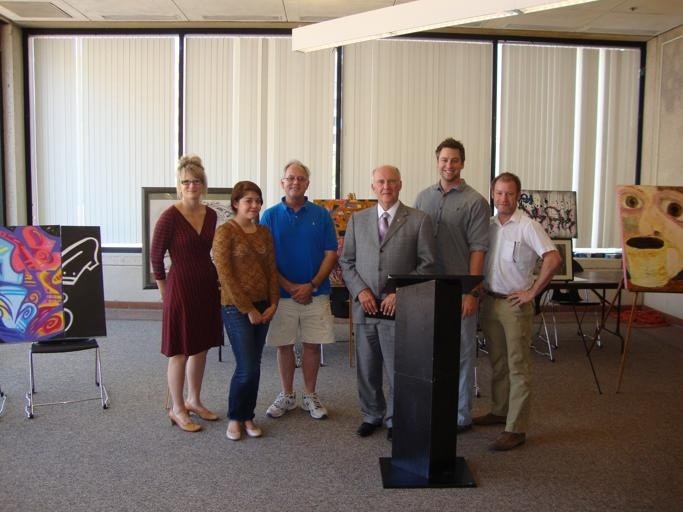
[{"xmin": 548, "ymin": 270, "xmax": 625, "ymax": 356}]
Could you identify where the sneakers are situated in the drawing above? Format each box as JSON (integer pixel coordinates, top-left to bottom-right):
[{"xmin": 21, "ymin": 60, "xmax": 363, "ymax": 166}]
[
  {"xmin": 266, "ymin": 390, "xmax": 297, "ymax": 418},
  {"xmin": 300, "ymin": 393, "xmax": 328, "ymax": 419}
]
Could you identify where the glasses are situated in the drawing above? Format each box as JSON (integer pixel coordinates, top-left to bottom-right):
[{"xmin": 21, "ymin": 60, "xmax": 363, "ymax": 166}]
[
  {"xmin": 180, "ymin": 179, "xmax": 203, "ymax": 185},
  {"xmin": 283, "ymin": 174, "xmax": 307, "ymax": 182}
]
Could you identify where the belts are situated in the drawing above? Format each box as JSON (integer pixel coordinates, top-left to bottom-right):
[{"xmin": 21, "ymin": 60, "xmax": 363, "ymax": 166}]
[{"xmin": 484, "ymin": 288, "xmax": 529, "ymax": 301}]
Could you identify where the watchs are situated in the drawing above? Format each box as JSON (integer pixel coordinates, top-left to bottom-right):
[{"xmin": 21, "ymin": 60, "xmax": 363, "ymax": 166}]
[
  {"xmin": 306, "ymin": 280, "xmax": 318, "ymax": 293},
  {"xmin": 467, "ymin": 290, "xmax": 481, "ymax": 297}
]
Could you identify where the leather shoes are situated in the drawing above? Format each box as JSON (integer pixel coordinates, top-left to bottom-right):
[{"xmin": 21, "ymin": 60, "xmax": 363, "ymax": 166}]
[
  {"xmin": 456, "ymin": 422, "xmax": 474, "ymax": 433},
  {"xmin": 474, "ymin": 411, "xmax": 508, "ymax": 425},
  {"xmin": 226, "ymin": 414, "xmax": 262, "ymax": 440},
  {"xmin": 387, "ymin": 421, "xmax": 402, "ymax": 440},
  {"xmin": 358, "ymin": 413, "xmax": 382, "ymax": 437},
  {"xmin": 487, "ymin": 430, "xmax": 526, "ymax": 449}
]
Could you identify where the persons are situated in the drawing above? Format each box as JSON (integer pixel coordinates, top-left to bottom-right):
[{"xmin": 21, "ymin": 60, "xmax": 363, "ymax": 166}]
[
  {"xmin": 472, "ymin": 171, "xmax": 563, "ymax": 450},
  {"xmin": 212, "ymin": 180, "xmax": 280, "ymax": 441},
  {"xmin": 256, "ymin": 158, "xmax": 338, "ymax": 421},
  {"xmin": 412, "ymin": 137, "xmax": 490, "ymax": 434},
  {"xmin": 149, "ymin": 156, "xmax": 222, "ymax": 433},
  {"xmin": 337, "ymin": 164, "xmax": 437, "ymax": 439}
]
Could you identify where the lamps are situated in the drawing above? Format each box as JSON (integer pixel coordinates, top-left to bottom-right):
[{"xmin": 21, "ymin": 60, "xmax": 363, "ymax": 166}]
[{"xmin": 286, "ymin": 0, "xmax": 598, "ymax": 55}]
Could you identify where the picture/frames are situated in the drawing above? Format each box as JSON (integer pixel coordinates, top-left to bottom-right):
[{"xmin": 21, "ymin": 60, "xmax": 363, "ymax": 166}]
[
  {"xmin": 139, "ymin": 185, "xmax": 260, "ymax": 291},
  {"xmin": 531, "ymin": 236, "xmax": 574, "ymax": 282}
]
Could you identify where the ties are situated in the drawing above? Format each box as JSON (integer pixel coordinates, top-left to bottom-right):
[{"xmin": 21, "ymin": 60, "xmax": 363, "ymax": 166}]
[{"xmin": 378, "ymin": 211, "xmax": 390, "ymax": 242}]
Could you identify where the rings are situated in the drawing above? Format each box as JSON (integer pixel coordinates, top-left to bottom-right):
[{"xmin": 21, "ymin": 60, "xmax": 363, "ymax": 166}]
[{"xmin": 388, "ymin": 306, "xmax": 392, "ymax": 309}]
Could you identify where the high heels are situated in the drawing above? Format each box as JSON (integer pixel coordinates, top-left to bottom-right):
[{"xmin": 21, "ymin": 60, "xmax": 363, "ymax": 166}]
[{"xmin": 168, "ymin": 401, "xmax": 219, "ymax": 431}]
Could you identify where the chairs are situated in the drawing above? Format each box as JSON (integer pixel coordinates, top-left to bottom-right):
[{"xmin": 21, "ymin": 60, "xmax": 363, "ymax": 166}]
[
  {"xmin": 535, "ymin": 258, "xmax": 601, "ymax": 350},
  {"xmin": 23, "ymin": 336, "xmax": 110, "ymax": 419}
]
